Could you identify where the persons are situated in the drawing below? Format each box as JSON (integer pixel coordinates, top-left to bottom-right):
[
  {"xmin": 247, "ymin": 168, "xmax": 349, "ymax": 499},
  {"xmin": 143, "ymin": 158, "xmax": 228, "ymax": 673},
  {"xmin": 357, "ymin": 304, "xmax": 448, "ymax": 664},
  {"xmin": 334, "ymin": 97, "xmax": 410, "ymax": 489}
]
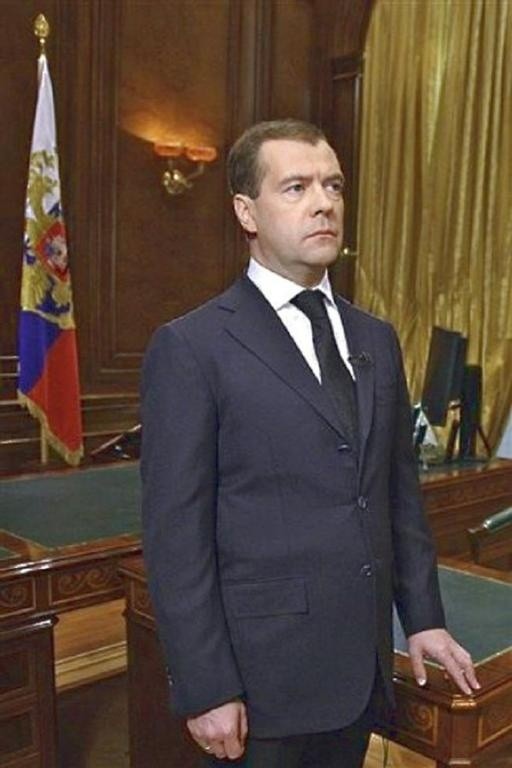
[{"xmin": 138, "ymin": 118, "xmax": 482, "ymax": 767}]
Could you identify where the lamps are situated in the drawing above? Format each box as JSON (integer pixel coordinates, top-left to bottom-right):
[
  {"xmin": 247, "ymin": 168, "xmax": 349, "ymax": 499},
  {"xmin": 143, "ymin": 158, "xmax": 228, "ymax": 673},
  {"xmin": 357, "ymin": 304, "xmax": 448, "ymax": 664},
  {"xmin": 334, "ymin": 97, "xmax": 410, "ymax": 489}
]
[{"xmin": 154, "ymin": 140, "xmax": 217, "ymax": 197}]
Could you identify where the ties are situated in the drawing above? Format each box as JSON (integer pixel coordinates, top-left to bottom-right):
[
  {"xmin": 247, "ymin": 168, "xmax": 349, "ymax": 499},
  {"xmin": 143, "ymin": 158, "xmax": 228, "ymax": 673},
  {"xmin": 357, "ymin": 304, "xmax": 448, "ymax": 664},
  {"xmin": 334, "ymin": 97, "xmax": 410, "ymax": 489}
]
[{"xmin": 290, "ymin": 290, "xmax": 360, "ymax": 443}]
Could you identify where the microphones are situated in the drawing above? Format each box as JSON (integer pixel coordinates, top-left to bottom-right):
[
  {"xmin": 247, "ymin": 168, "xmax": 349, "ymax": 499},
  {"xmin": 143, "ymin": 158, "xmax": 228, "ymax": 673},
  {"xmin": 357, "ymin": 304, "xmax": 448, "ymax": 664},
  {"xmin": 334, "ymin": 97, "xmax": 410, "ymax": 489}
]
[
  {"xmin": 481, "ymin": 504, "xmax": 511, "ymax": 532},
  {"xmin": 90, "ymin": 414, "xmax": 144, "ymax": 458}
]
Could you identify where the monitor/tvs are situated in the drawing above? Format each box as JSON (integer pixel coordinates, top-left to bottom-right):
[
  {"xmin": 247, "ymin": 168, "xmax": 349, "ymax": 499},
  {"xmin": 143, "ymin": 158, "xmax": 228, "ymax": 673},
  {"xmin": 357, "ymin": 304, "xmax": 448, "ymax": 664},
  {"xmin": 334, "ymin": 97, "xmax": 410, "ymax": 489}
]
[{"xmin": 424, "ymin": 327, "xmax": 490, "ymax": 463}]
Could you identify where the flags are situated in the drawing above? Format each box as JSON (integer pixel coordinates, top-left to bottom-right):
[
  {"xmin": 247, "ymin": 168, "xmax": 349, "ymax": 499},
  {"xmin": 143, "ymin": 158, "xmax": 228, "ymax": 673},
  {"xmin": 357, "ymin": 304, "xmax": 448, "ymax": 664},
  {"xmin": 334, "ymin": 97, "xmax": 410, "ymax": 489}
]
[{"xmin": 14, "ymin": 13, "xmax": 86, "ymax": 467}]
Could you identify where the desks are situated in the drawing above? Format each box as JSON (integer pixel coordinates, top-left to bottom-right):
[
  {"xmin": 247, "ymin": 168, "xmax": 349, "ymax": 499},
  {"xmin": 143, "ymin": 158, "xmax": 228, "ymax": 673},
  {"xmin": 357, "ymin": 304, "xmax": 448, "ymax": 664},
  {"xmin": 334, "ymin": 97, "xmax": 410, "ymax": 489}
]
[{"xmin": 0, "ymin": 442, "xmax": 512, "ymax": 768}]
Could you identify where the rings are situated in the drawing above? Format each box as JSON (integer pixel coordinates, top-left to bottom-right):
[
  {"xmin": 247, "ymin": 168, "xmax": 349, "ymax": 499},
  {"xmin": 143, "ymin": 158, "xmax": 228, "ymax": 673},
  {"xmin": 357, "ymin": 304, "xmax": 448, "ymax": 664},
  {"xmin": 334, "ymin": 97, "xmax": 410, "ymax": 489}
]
[{"xmin": 203, "ymin": 746, "xmax": 210, "ymax": 750}]
